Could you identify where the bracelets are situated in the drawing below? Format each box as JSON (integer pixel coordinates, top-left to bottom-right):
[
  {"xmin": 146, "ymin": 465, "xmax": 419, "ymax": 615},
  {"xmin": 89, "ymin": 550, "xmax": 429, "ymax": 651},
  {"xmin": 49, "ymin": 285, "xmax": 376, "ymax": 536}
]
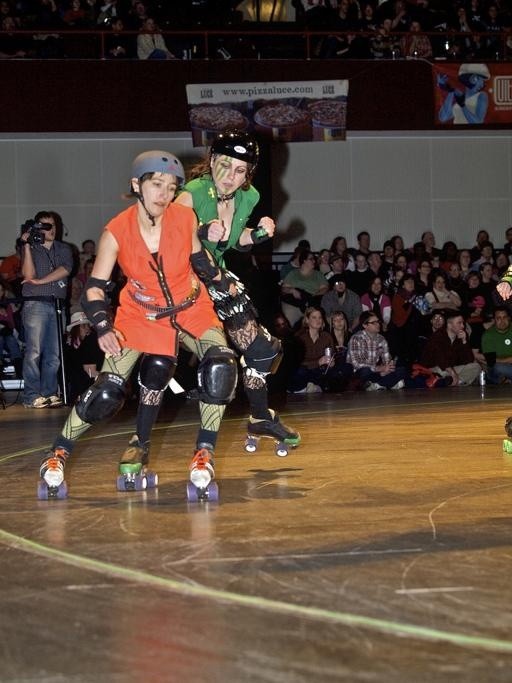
[{"xmin": 247, "ymin": 224, "xmax": 269, "ymax": 248}]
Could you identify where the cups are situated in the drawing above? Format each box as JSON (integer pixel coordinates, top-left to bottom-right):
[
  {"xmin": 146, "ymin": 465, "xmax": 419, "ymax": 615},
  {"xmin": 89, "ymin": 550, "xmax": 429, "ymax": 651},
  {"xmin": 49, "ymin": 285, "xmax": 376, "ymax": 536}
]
[{"xmin": 324, "ymin": 344, "xmax": 332, "ymax": 356}]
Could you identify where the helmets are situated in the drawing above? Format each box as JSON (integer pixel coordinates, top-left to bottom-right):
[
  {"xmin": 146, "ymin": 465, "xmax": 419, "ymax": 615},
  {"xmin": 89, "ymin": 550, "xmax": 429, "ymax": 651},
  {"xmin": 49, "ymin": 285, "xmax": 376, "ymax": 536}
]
[
  {"xmin": 128, "ymin": 151, "xmax": 185, "ymax": 194},
  {"xmin": 208, "ymin": 129, "xmax": 260, "ymax": 168}
]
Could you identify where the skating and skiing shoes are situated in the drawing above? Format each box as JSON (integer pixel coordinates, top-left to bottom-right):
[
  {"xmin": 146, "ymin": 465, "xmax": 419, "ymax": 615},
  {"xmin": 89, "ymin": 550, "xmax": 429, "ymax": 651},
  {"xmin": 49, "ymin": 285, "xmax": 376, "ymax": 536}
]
[
  {"xmin": 243, "ymin": 408, "xmax": 301, "ymax": 457},
  {"xmin": 37, "ymin": 445, "xmax": 72, "ymax": 502},
  {"xmin": 116, "ymin": 435, "xmax": 159, "ymax": 493},
  {"xmin": 186, "ymin": 447, "xmax": 219, "ymax": 503},
  {"xmin": 499, "ymin": 416, "xmax": 512, "ymax": 454}
]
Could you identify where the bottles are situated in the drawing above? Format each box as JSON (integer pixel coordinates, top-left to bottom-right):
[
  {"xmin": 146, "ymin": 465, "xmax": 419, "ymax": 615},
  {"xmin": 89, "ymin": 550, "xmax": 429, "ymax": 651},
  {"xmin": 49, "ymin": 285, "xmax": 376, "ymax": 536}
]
[{"xmin": 479, "ymin": 367, "xmax": 486, "ymax": 385}]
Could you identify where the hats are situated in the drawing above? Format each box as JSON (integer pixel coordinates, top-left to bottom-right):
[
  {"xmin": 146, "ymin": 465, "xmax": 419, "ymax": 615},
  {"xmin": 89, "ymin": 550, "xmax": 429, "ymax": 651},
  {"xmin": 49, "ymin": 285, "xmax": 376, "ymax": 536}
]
[
  {"xmin": 329, "ymin": 253, "xmax": 344, "ymax": 265},
  {"xmin": 458, "ymin": 63, "xmax": 491, "ymax": 80},
  {"xmin": 66, "ymin": 312, "xmax": 95, "ymax": 332}
]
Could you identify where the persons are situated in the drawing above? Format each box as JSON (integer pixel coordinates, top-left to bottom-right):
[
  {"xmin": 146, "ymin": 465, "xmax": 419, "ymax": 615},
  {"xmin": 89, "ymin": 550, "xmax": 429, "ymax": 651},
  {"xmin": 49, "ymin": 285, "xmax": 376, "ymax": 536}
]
[
  {"xmin": 113, "ymin": 126, "xmax": 302, "ymax": 479},
  {"xmin": 497, "ymin": 265, "xmax": 510, "ymax": 440},
  {"xmin": 13, "ymin": 210, "xmax": 75, "ymax": 411},
  {"xmin": 291, "ymin": 0, "xmax": 511, "ymax": 63},
  {"xmin": 37, "ymin": 149, "xmax": 240, "ymax": 491},
  {"xmin": 0, "ymin": 240, "xmax": 280, "ymax": 410},
  {"xmin": 431, "ymin": 58, "xmax": 495, "ymax": 127},
  {"xmin": 1, "ymin": 1, "xmax": 177, "ymax": 63},
  {"xmin": 242, "ymin": 227, "xmax": 511, "ymax": 394}
]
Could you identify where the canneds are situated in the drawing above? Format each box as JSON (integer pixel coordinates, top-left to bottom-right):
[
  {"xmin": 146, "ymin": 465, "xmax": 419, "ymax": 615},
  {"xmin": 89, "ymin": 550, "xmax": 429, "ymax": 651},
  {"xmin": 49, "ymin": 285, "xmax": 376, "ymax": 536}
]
[
  {"xmin": 479, "ymin": 370, "xmax": 486, "ymax": 386},
  {"xmin": 325, "ymin": 347, "xmax": 332, "ymax": 357},
  {"xmin": 182, "ymin": 50, "xmax": 188, "ymax": 60},
  {"xmin": 479, "ymin": 387, "xmax": 486, "ymax": 400}
]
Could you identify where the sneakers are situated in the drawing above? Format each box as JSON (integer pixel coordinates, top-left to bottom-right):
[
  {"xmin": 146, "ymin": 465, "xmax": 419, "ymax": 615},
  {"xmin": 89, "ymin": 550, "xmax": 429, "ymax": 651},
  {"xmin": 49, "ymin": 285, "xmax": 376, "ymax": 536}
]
[
  {"xmin": 296, "ymin": 374, "xmax": 512, "ymax": 394},
  {"xmin": 23, "ymin": 392, "xmax": 64, "ymax": 408},
  {"xmin": 183, "ymin": 385, "xmax": 202, "ymax": 401}
]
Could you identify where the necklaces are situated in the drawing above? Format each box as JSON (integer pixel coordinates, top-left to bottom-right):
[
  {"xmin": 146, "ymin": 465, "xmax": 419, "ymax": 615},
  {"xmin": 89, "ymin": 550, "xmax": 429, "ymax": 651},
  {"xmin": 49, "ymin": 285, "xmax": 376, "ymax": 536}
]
[{"xmin": 216, "ymin": 191, "xmax": 232, "ymax": 209}]
